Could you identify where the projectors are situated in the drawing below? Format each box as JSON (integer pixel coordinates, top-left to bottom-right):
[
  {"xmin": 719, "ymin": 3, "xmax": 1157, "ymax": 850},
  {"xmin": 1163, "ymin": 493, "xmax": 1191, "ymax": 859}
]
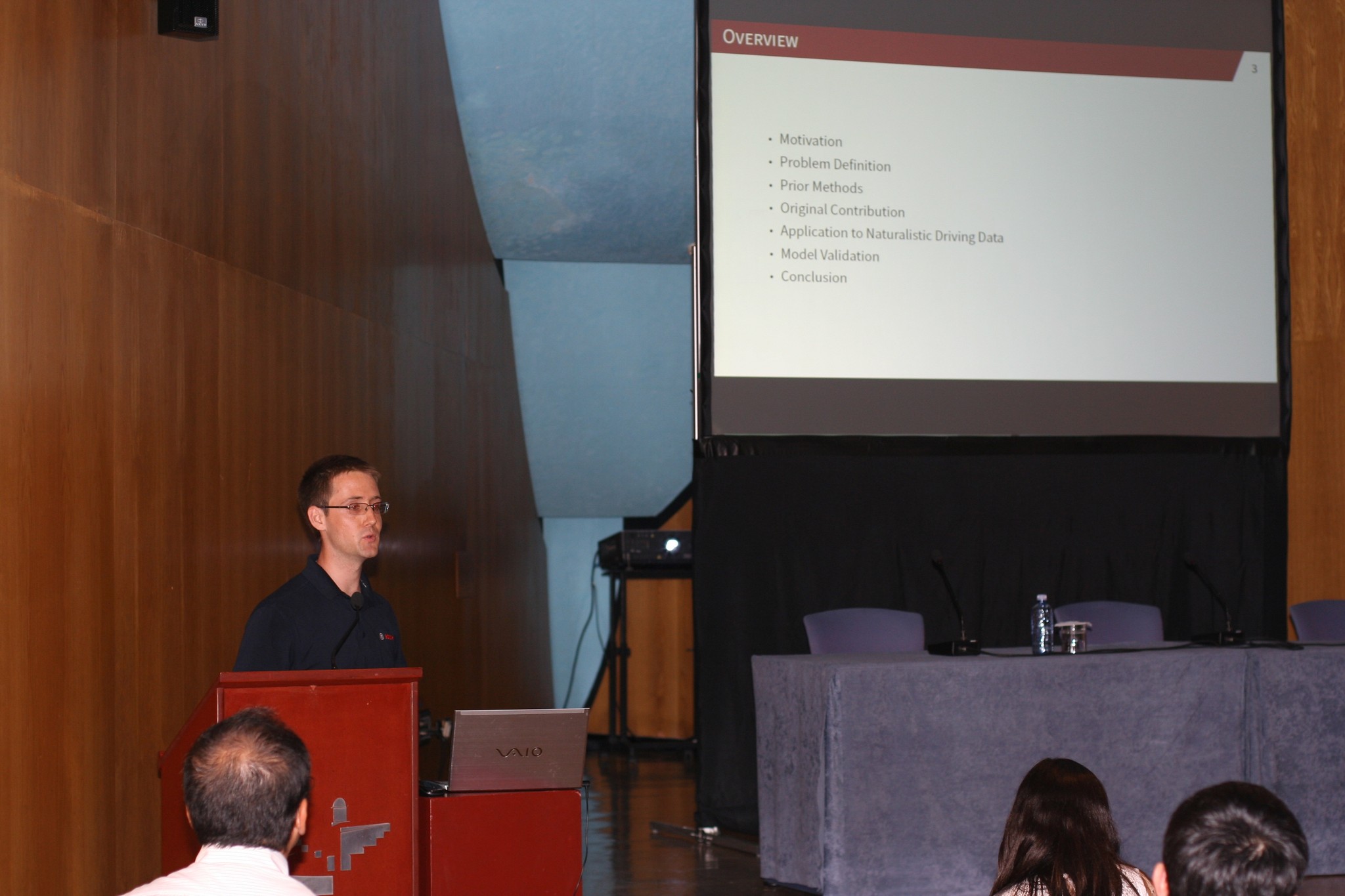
[{"xmin": 598, "ymin": 527, "xmax": 694, "ymax": 569}]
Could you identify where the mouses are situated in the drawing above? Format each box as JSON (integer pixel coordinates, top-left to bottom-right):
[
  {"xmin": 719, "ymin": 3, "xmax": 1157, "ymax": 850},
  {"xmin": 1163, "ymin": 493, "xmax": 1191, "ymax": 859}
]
[{"xmin": 419, "ymin": 779, "xmax": 446, "ymax": 796}]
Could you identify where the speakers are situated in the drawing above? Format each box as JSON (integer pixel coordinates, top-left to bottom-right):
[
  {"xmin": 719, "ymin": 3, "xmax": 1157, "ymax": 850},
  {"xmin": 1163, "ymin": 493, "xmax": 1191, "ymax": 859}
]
[{"xmin": 156, "ymin": 0, "xmax": 220, "ymax": 43}]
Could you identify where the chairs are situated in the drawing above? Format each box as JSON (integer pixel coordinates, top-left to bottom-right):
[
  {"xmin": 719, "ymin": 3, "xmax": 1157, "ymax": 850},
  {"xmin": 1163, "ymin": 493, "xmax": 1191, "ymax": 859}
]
[
  {"xmin": 804, "ymin": 606, "xmax": 926, "ymax": 655},
  {"xmin": 1289, "ymin": 599, "xmax": 1345, "ymax": 643},
  {"xmin": 1054, "ymin": 602, "xmax": 1164, "ymax": 648}
]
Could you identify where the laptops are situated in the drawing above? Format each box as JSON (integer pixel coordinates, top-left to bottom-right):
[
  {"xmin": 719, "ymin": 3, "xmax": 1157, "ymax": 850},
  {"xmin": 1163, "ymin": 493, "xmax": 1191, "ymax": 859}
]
[{"xmin": 421, "ymin": 708, "xmax": 591, "ymax": 792}]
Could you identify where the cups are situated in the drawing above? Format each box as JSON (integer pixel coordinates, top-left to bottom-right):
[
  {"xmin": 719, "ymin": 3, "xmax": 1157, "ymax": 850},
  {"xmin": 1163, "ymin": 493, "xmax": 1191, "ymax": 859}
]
[{"xmin": 1060, "ymin": 623, "xmax": 1086, "ymax": 653}]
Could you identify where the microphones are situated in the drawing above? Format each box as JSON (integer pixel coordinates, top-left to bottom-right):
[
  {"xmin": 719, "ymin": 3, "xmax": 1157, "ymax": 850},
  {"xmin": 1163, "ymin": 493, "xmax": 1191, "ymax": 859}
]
[
  {"xmin": 331, "ymin": 591, "xmax": 365, "ymax": 670},
  {"xmin": 1187, "ymin": 558, "xmax": 1238, "ymax": 645},
  {"xmin": 927, "ymin": 547, "xmax": 983, "ymax": 657}
]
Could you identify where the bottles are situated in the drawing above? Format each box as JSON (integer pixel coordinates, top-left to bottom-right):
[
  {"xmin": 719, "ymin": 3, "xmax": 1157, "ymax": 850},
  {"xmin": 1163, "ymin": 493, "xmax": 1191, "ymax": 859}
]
[{"xmin": 1031, "ymin": 594, "xmax": 1054, "ymax": 654}]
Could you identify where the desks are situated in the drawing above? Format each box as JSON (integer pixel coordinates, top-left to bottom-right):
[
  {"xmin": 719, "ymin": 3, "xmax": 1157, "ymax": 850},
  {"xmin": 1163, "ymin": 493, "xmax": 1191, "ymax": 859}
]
[
  {"xmin": 601, "ymin": 569, "xmax": 702, "ymax": 784},
  {"xmin": 750, "ymin": 643, "xmax": 1345, "ymax": 896}
]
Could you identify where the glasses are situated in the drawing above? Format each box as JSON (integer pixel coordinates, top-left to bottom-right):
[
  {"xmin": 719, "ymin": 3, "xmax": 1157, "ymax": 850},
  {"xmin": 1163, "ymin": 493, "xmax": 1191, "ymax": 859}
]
[{"xmin": 317, "ymin": 501, "xmax": 391, "ymax": 515}]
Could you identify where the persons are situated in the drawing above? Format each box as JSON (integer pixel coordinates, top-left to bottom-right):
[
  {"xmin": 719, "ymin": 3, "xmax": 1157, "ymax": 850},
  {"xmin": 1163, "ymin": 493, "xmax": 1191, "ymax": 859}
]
[
  {"xmin": 1152, "ymin": 780, "xmax": 1310, "ymax": 896},
  {"xmin": 231, "ymin": 453, "xmax": 408, "ymax": 670},
  {"xmin": 119, "ymin": 703, "xmax": 322, "ymax": 896},
  {"xmin": 988, "ymin": 759, "xmax": 1156, "ymax": 896}
]
